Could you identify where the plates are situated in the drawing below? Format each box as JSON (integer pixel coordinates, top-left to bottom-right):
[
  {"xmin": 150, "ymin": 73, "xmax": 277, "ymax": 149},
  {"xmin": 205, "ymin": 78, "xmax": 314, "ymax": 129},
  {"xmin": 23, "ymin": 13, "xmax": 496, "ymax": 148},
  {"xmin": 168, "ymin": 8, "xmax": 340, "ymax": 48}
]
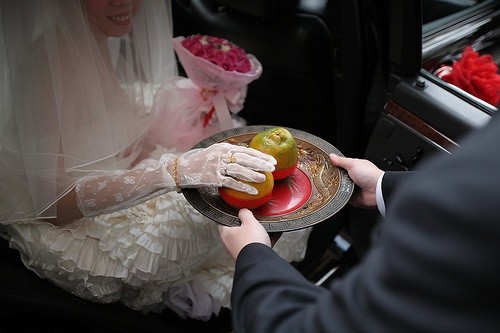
[{"xmin": 180, "ymin": 125, "xmax": 355, "ymax": 232}]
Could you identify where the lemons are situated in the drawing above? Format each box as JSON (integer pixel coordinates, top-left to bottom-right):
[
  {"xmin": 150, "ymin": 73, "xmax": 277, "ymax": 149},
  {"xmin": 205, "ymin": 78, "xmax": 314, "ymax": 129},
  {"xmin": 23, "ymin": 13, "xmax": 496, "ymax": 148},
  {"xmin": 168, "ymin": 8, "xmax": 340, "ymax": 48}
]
[
  {"xmin": 219, "ymin": 172, "xmax": 274, "ymax": 211},
  {"xmin": 248, "ymin": 128, "xmax": 298, "ymax": 180}
]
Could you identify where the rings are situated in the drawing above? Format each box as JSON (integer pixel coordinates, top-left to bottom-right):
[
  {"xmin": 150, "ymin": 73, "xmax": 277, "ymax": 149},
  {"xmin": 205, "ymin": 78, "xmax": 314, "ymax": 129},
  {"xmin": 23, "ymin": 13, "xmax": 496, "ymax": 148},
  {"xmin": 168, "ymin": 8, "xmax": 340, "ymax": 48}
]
[
  {"xmin": 229, "ymin": 154, "xmax": 235, "ymax": 162},
  {"xmin": 225, "ymin": 164, "xmax": 227, "ymax": 175}
]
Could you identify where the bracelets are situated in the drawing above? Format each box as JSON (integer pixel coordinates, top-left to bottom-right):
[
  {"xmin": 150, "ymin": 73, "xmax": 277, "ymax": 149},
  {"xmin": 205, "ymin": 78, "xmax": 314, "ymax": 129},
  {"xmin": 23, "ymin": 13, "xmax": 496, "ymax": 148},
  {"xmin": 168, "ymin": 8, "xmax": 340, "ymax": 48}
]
[{"xmin": 173, "ymin": 155, "xmax": 181, "ymax": 193}]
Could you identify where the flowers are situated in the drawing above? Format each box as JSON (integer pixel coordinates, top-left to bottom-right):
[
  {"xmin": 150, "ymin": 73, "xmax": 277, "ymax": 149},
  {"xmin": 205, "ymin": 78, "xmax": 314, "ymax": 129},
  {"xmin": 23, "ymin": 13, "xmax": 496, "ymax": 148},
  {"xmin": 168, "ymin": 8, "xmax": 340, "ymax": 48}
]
[
  {"xmin": 172, "ymin": 34, "xmax": 262, "ymax": 146},
  {"xmin": 437, "ymin": 46, "xmax": 500, "ymax": 111}
]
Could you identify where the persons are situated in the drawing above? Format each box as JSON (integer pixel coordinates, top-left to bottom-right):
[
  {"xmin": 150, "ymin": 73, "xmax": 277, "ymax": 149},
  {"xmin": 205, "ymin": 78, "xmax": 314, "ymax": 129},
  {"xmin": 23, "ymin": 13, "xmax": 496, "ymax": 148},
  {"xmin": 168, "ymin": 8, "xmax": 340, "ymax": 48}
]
[
  {"xmin": 1, "ymin": 0, "xmax": 279, "ymax": 322},
  {"xmin": 217, "ymin": 95, "xmax": 499, "ymax": 332}
]
